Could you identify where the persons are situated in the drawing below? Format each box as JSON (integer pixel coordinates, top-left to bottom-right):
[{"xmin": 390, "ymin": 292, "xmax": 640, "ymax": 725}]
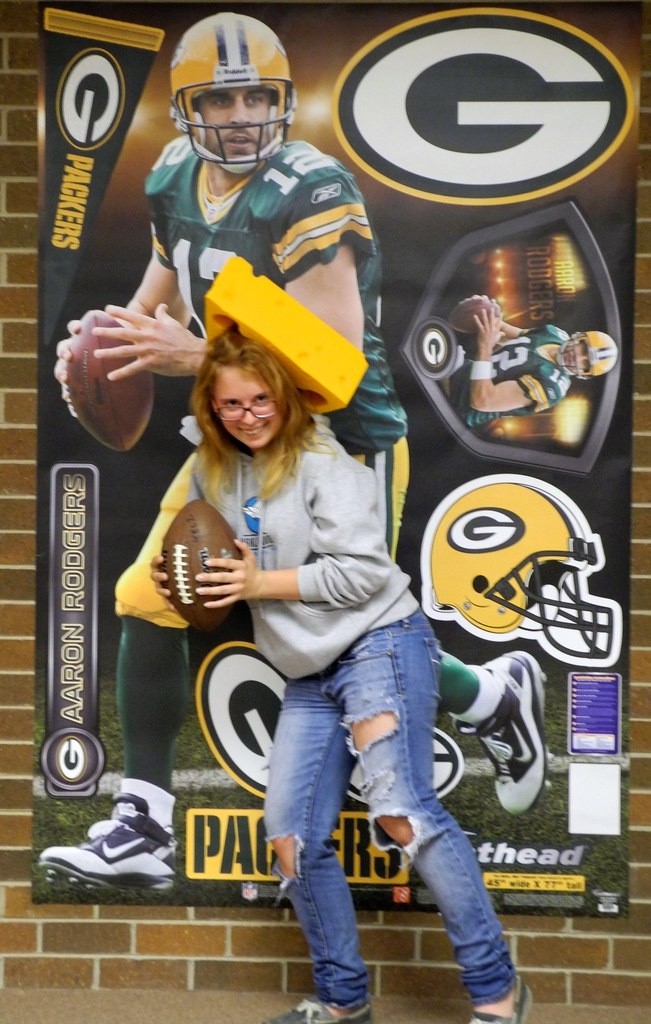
[
  {"xmin": 428, "ymin": 292, "xmax": 621, "ymax": 428},
  {"xmin": 32, "ymin": 7, "xmax": 549, "ymax": 895},
  {"xmin": 149, "ymin": 322, "xmax": 532, "ymax": 1024}
]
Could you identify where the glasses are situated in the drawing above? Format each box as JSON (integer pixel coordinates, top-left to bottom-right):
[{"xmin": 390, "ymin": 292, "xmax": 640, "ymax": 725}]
[{"xmin": 211, "ymin": 395, "xmax": 277, "ymax": 421}]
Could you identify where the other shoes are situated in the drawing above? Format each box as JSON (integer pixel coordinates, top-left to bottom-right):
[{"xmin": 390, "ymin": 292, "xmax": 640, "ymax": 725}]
[
  {"xmin": 466, "ymin": 977, "xmax": 533, "ymax": 1023},
  {"xmin": 262, "ymin": 996, "xmax": 371, "ymax": 1023}
]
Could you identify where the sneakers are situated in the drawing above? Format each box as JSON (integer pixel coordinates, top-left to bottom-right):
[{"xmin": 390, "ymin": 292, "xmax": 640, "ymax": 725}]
[
  {"xmin": 38, "ymin": 792, "xmax": 175, "ymax": 895},
  {"xmin": 480, "ymin": 651, "xmax": 544, "ymax": 817}
]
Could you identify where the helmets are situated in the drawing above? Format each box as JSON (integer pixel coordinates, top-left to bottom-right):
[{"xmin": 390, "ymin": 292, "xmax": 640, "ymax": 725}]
[
  {"xmin": 558, "ymin": 330, "xmax": 619, "ymax": 379},
  {"xmin": 172, "ymin": 12, "xmax": 297, "ymax": 169}
]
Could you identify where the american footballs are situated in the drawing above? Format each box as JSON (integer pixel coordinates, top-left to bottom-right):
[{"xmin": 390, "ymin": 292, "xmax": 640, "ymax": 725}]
[
  {"xmin": 448, "ymin": 298, "xmax": 501, "ymax": 332},
  {"xmin": 66, "ymin": 308, "xmax": 153, "ymax": 451},
  {"xmin": 158, "ymin": 498, "xmax": 242, "ymax": 630}
]
